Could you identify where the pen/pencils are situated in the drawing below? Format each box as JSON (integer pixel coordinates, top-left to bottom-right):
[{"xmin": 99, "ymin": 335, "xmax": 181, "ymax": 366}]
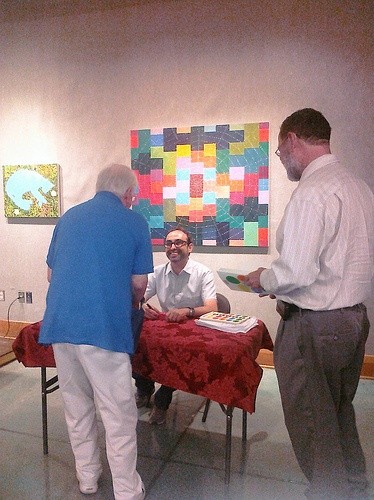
[{"xmin": 146, "ymin": 303, "xmax": 160, "ymax": 315}]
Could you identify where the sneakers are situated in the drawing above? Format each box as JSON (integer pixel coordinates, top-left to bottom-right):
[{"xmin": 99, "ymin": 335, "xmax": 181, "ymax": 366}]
[
  {"xmin": 149, "ymin": 404, "xmax": 167, "ymax": 425},
  {"xmin": 135, "ymin": 386, "xmax": 155, "ymax": 408}
]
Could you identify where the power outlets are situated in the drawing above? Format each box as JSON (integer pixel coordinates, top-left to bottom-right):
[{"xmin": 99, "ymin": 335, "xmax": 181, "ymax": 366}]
[{"xmin": 18, "ymin": 291, "xmax": 25, "ymax": 303}]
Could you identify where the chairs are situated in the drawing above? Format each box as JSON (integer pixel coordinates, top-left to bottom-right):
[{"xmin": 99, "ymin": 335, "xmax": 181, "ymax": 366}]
[{"xmin": 146, "ymin": 293, "xmax": 231, "ymax": 422}]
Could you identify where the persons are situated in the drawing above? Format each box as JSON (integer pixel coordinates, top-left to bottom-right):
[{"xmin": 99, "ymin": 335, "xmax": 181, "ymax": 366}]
[
  {"xmin": 135, "ymin": 227, "xmax": 218, "ymax": 424},
  {"xmin": 245, "ymin": 108, "xmax": 374, "ymax": 500},
  {"xmin": 38, "ymin": 163, "xmax": 154, "ymax": 500}
]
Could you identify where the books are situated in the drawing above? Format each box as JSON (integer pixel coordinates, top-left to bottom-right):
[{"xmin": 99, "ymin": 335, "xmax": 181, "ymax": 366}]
[
  {"xmin": 199, "ymin": 311, "xmax": 252, "ymax": 325},
  {"xmin": 216, "ymin": 267, "xmax": 270, "ymax": 294}
]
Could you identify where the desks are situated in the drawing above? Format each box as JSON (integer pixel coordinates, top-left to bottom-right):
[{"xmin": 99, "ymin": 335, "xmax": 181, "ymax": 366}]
[{"xmin": 11, "ymin": 312, "xmax": 274, "ymax": 484}]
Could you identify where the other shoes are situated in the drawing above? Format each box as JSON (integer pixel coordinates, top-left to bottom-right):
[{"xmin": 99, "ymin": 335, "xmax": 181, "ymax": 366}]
[
  {"xmin": 141, "ymin": 481, "xmax": 146, "ymax": 499},
  {"xmin": 79, "ymin": 482, "xmax": 98, "ymax": 494}
]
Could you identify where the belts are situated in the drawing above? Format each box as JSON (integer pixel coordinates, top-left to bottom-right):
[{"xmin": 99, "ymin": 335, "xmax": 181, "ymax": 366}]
[{"xmin": 288, "ymin": 304, "xmax": 312, "ymax": 313}]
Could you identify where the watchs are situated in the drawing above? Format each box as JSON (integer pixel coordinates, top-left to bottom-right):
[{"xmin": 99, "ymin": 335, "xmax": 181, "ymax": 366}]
[{"xmin": 184, "ymin": 306, "xmax": 195, "ymax": 317}]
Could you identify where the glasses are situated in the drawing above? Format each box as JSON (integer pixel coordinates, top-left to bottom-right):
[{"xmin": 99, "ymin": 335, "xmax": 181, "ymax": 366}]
[
  {"xmin": 275, "ymin": 136, "xmax": 289, "ymax": 157},
  {"xmin": 128, "ymin": 192, "xmax": 136, "ymax": 201},
  {"xmin": 164, "ymin": 240, "xmax": 188, "ymax": 248}
]
